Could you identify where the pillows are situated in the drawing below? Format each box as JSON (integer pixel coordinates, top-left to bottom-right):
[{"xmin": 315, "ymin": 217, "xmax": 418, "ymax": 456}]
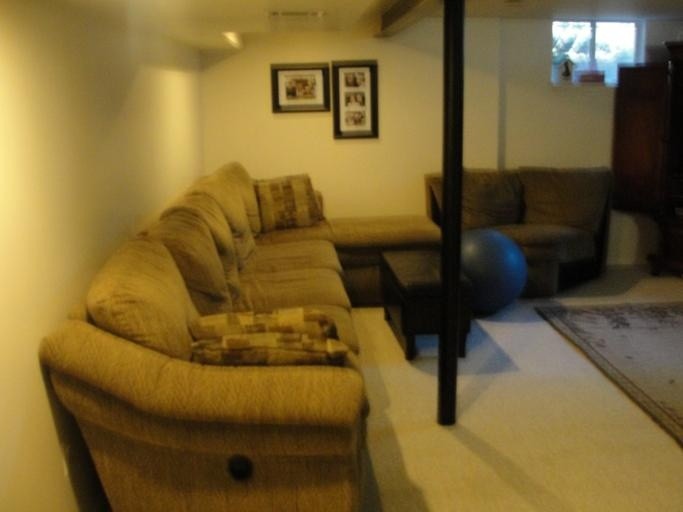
[
  {"xmin": 158, "ymin": 193, "xmax": 242, "ymax": 298},
  {"xmin": 213, "ymin": 160, "xmax": 264, "ymax": 243},
  {"xmin": 83, "ymin": 232, "xmax": 200, "ymax": 359},
  {"xmin": 253, "ymin": 171, "xmax": 329, "ymax": 229},
  {"xmin": 190, "ymin": 174, "xmax": 258, "ymax": 264},
  {"xmin": 190, "ymin": 336, "xmax": 349, "ymax": 367},
  {"xmin": 187, "ymin": 309, "xmax": 341, "ymax": 341},
  {"xmin": 146, "ymin": 207, "xmax": 234, "ymax": 316}
]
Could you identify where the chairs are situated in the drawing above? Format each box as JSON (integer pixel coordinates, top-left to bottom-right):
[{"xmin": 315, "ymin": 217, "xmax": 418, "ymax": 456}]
[{"xmin": 423, "ymin": 165, "xmax": 612, "ymax": 298}]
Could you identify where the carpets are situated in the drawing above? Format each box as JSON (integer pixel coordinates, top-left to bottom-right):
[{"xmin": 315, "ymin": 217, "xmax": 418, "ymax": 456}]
[{"xmin": 533, "ymin": 301, "xmax": 682, "ymax": 450}]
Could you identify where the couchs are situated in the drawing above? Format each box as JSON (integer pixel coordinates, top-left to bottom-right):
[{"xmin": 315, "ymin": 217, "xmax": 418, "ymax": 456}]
[{"xmin": 36, "ymin": 161, "xmax": 441, "ymax": 511}]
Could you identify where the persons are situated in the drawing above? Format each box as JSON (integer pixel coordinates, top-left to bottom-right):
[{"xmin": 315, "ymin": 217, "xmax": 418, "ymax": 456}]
[
  {"xmin": 285, "ymin": 78, "xmax": 317, "ymax": 99},
  {"xmin": 344, "ymin": 71, "xmax": 366, "ymax": 126}
]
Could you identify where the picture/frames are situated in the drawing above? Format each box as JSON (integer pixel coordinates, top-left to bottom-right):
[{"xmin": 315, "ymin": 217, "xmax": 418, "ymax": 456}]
[{"xmin": 269, "ymin": 60, "xmax": 379, "ymax": 141}]
